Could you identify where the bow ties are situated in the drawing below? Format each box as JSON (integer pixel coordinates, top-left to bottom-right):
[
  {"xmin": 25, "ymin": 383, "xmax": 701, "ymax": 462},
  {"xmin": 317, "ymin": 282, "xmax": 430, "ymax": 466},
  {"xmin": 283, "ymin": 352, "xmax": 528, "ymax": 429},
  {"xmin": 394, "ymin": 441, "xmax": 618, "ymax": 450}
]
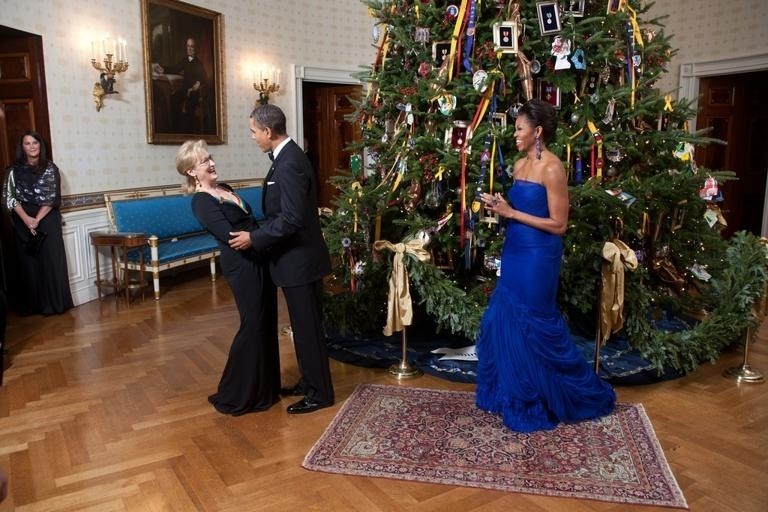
[{"xmin": 266, "ymin": 151, "xmax": 277, "ymax": 162}]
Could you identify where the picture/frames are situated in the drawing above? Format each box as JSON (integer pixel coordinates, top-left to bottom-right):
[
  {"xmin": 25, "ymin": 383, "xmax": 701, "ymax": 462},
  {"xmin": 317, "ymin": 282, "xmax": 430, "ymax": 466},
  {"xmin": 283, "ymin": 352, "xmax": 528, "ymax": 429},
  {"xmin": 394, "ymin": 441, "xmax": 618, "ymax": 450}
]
[
  {"xmin": 536, "ymin": 2, "xmax": 562, "ymax": 36},
  {"xmin": 492, "ymin": 112, "xmax": 507, "ymax": 128},
  {"xmin": 493, "ymin": 21, "xmax": 518, "ymax": 54},
  {"xmin": 432, "ymin": 40, "xmax": 450, "ymax": 69},
  {"xmin": 445, "ymin": 121, "xmax": 472, "ymax": 155},
  {"xmin": 671, "ymin": 201, "xmax": 687, "ymax": 231},
  {"xmin": 562, "ymin": 0, "xmax": 585, "ymax": 17},
  {"xmin": 537, "ymin": 76, "xmax": 562, "ymax": 110},
  {"xmin": 580, "ymin": 68, "xmax": 601, "ymax": 97},
  {"xmin": 480, "ymin": 200, "xmax": 499, "ymax": 224},
  {"xmin": 141, "ymin": 0, "xmax": 225, "ymax": 144}
]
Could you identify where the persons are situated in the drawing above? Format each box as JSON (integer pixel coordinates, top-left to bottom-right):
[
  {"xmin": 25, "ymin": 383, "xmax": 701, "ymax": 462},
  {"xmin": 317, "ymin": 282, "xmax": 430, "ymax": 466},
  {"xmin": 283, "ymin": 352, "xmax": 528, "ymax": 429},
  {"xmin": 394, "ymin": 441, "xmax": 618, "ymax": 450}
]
[
  {"xmin": 174, "ymin": 137, "xmax": 283, "ymax": 417},
  {"xmin": 152, "ymin": 37, "xmax": 207, "ymax": 117},
  {"xmin": 474, "ymin": 99, "xmax": 617, "ymax": 433},
  {"xmin": 0, "ymin": 128, "xmax": 75, "ymax": 317},
  {"xmin": 227, "ymin": 104, "xmax": 336, "ymax": 414}
]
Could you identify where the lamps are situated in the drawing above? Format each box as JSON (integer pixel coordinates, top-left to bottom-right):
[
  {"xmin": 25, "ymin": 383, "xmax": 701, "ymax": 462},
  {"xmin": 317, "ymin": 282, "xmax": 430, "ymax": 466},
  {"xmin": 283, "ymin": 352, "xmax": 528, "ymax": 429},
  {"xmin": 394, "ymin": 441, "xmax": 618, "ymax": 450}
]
[
  {"xmin": 253, "ymin": 67, "xmax": 280, "ymax": 107},
  {"xmin": 91, "ymin": 33, "xmax": 129, "ymax": 112}
]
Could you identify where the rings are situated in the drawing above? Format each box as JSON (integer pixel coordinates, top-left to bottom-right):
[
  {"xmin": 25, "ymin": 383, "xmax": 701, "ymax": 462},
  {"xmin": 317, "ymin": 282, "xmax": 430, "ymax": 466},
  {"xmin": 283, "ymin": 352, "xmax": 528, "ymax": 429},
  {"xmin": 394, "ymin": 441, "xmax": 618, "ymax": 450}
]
[{"xmin": 491, "ymin": 198, "xmax": 497, "ymax": 204}]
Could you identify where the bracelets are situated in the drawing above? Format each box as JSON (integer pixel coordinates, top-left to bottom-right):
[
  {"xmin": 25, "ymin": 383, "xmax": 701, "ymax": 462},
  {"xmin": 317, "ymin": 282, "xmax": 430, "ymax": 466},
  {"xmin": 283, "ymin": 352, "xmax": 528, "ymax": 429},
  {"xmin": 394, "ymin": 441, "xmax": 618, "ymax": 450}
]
[{"xmin": 509, "ymin": 208, "xmax": 515, "ymax": 220}]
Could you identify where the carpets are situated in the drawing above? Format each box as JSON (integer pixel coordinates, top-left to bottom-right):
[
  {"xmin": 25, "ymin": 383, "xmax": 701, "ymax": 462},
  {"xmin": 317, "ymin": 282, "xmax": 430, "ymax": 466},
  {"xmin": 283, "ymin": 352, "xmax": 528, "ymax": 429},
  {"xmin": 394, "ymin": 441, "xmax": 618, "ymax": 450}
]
[{"xmin": 301, "ymin": 383, "xmax": 689, "ymax": 509}]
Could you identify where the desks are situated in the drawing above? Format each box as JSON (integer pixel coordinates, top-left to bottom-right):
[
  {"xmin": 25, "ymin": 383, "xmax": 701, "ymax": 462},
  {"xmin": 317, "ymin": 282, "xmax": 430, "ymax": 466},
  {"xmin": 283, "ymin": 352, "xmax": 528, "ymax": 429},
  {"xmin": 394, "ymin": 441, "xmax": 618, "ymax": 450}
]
[{"xmin": 154, "ymin": 74, "xmax": 185, "ymax": 133}]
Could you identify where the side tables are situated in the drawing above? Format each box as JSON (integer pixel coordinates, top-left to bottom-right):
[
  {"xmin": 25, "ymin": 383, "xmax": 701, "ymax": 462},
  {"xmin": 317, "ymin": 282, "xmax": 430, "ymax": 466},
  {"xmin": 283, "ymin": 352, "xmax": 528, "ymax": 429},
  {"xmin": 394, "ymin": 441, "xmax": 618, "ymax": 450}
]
[{"xmin": 91, "ymin": 233, "xmax": 148, "ymax": 305}]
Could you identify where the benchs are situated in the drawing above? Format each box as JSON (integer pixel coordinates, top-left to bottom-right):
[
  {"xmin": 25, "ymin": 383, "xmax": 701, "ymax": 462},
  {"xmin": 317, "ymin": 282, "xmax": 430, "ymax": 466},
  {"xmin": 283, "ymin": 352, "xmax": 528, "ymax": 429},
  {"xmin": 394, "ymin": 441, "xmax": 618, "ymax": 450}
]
[{"xmin": 104, "ymin": 179, "xmax": 264, "ymax": 300}]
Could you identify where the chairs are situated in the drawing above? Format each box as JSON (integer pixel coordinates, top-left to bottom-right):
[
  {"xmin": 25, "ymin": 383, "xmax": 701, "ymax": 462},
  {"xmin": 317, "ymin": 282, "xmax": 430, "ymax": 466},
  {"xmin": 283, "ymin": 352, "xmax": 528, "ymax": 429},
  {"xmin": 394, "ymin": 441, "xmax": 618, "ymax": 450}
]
[{"xmin": 178, "ymin": 84, "xmax": 211, "ymax": 134}]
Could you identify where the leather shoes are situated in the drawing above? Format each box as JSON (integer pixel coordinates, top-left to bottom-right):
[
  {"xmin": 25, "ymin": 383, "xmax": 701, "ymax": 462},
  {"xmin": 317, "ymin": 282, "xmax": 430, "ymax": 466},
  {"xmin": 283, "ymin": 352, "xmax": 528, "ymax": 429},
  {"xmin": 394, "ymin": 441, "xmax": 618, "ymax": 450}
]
[
  {"xmin": 285, "ymin": 395, "xmax": 334, "ymax": 415},
  {"xmin": 279, "ymin": 382, "xmax": 305, "ymax": 398}
]
